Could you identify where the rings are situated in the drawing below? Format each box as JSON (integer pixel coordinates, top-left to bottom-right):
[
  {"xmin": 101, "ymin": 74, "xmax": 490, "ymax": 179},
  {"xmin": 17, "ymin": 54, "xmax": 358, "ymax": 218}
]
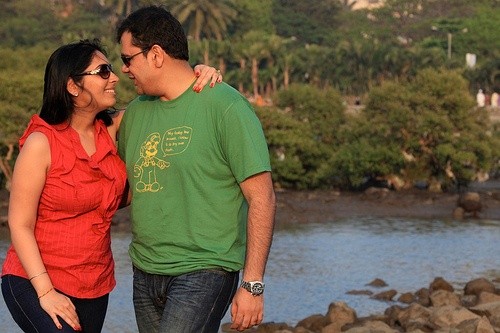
[{"xmin": 216, "ymin": 69, "xmax": 221, "ymax": 76}]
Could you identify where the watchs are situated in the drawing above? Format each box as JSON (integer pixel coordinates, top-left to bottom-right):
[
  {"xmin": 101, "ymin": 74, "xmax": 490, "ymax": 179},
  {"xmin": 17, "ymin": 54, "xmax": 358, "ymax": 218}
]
[{"xmin": 240, "ymin": 280, "xmax": 265, "ymax": 297}]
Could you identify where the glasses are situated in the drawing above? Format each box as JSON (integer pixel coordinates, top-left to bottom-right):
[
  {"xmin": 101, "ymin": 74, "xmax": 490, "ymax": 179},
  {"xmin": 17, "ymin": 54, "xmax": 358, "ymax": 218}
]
[
  {"xmin": 72, "ymin": 64, "xmax": 113, "ymax": 79},
  {"xmin": 121, "ymin": 48, "xmax": 150, "ymax": 68}
]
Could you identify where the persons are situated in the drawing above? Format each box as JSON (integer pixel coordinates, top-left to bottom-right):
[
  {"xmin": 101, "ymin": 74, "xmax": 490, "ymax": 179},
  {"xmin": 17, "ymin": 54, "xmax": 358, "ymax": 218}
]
[
  {"xmin": 0, "ymin": 38, "xmax": 222, "ymax": 333},
  {"xmin": 476, "ymin": 88, "xmax": 500, "ymax": 111},
  {"xmin": 117, "ymin": 5, "xmax": 276, "ymax": 333}
]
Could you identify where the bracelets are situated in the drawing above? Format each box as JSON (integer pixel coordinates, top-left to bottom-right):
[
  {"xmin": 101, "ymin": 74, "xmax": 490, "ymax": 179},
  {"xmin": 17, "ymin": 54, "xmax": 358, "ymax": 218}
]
[
  {"xmin": 38, "ymin": 288, "xmax": 54, "ymax": 299},
  {"xmin": 29, "ymin": 272, "xmax": 48, "ymax": 281}
]
